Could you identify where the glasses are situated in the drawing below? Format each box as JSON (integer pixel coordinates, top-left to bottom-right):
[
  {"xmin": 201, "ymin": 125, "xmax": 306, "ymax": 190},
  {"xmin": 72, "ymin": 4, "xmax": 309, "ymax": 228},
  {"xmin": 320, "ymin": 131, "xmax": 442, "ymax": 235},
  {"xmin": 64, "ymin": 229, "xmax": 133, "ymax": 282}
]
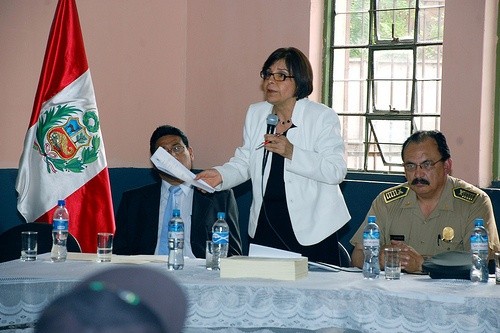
[
  {"xmin": 163, "ymin": 145, "xmax": 188, "ymax": 153},
  {"xmin": 403, "ymin": 158, "xmax": 442, "ymax": 172},
  {"xmin": 259, "ymin": 70, "xmax": 294, "ymax": 82}
]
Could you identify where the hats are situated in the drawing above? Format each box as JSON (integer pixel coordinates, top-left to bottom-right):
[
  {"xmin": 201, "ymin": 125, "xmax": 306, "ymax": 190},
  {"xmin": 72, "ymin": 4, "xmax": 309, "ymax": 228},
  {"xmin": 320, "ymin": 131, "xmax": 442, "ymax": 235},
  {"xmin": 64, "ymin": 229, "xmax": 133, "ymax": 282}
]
[{"xmin": 33, "ymin": 266, "xmax": 186, "ymax": 333}]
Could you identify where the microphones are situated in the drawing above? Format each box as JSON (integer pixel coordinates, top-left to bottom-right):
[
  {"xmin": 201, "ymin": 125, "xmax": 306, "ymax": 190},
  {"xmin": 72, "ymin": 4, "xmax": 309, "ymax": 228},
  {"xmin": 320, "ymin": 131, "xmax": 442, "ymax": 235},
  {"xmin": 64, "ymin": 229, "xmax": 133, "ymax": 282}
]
[{"xmin": 262, "ymin": 114, "xmax": 279, "ymax": 168}]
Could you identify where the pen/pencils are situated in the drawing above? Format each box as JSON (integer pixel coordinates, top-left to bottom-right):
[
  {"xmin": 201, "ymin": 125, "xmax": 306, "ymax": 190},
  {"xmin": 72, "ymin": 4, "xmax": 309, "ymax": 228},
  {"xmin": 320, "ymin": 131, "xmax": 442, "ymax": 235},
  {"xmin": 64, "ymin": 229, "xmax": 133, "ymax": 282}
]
[
  {"xmin": 437, "ymin": 234, "xmax": 441, "ymax": 246},
  {"xmin": 256, "ymin": 132, "xmax": 283, "ymax": 150}
]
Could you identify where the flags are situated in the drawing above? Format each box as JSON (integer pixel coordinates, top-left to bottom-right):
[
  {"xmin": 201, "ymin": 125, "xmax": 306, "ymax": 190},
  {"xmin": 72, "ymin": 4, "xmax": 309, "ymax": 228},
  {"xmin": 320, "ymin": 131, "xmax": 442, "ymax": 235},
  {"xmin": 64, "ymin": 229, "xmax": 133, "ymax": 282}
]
[{"xmin": 14, "ymin": 0, "xmax": 116, "ymax": 255}]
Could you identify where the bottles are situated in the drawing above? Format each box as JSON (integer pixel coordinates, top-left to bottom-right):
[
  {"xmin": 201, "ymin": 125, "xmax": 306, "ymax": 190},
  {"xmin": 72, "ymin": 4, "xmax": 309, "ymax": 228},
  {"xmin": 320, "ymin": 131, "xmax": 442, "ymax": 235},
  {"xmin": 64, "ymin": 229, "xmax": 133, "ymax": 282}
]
[
  {"xmin": 168, "ymin": 209, "xmax": 185, "ymax": 270},
  {"xmin": 362, "ymin": 216, "xmax": 381, "ymax": 279},
  {"xmin": 469, "ymin": 219, "xmax": 489, "ymax": 282},
  {"xmin": 50, "ymin": 200, "xmax": 69, "ymax": 262},
  {"xmin": 211, "ymin": 212, "xmax": 229, "ymax": 267}
]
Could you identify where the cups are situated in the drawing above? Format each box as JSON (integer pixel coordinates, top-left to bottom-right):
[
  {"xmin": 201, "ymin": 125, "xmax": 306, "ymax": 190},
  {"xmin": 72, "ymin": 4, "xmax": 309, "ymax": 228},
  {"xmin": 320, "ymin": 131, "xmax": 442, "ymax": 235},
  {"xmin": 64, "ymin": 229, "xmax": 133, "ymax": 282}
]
[
  {"xmin": 206, "ymin": 241, "xmax": 221, "ymax": 270},
  {"xmin": 384, "ymin": 248, "xmax": 402, "ymax": 280},
  {"xmin": 96, "ymin": 232, "xmax": 114, "ymax": 262},
  {"xmin": 494, "ymin": 252, "xmax": 500, "ymax": 285},
  {"xmin": 21, "ymin": 231, "xmax": 38, "ymax": 261}
]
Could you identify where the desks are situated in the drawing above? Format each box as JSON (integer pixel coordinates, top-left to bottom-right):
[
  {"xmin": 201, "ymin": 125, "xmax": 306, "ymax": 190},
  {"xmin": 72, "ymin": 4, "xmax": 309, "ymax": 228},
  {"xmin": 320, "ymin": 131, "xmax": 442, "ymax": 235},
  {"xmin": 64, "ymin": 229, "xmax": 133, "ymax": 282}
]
[{"xmin": 0, "ymin": 250, "xmax": 500, "ymax": 333}]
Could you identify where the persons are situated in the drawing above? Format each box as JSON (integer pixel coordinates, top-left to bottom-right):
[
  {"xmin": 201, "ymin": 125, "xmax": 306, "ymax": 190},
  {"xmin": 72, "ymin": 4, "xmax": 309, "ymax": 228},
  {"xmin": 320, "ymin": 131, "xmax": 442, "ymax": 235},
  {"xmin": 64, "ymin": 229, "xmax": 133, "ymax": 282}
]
[
  {"xmin": 349, "ymin": 129, "xmax": 500, "ymax": 276},
  {"xmin": 113, "ymin": 125, "xmax": 243, "ymax": 259},
  {"xmin": 195, "ymin": 47, "xmax": 351, "ymax": 269}
]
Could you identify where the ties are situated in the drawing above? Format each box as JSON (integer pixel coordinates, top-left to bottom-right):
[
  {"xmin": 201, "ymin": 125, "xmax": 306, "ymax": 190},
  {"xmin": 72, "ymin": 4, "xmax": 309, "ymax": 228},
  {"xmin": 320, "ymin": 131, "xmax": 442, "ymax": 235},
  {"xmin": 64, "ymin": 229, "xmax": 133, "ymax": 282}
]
[{"xmin": 159, "ymin": 186, "xmax": 181, "ymax": 255}]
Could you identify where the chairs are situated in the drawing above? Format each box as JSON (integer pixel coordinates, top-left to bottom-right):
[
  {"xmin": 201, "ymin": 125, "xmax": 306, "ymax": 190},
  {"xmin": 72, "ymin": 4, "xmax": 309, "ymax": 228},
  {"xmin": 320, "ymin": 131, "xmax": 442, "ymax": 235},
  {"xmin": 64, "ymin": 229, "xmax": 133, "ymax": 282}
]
[{"xmin": 0, "ymin": 222, "xmax": 82, "ymax": 263}]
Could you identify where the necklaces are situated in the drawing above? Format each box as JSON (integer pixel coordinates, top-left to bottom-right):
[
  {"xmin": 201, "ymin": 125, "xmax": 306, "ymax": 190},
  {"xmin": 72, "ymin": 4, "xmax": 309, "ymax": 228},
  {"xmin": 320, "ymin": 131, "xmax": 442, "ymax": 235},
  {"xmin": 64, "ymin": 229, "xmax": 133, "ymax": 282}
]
[{"xmin": 275, "ymin": 113, "xmax": 290, "ymax": 125}]
